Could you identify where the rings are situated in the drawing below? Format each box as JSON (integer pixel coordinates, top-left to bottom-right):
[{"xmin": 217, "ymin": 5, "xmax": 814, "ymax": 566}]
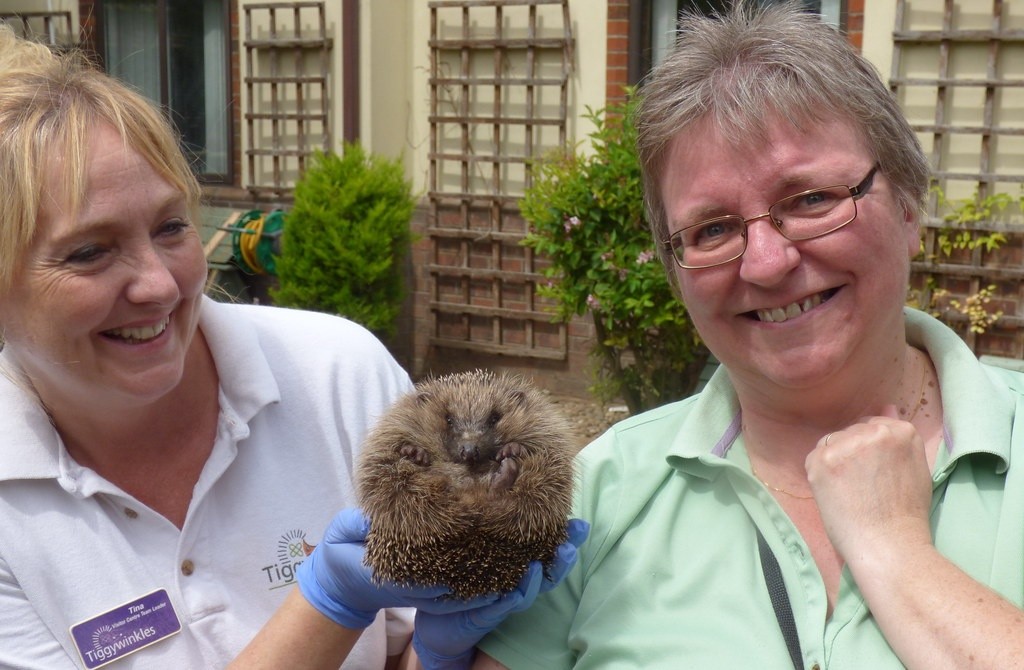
[{"xmin": 825, "ymin": 432, "xmax": 834, "ymax": 446}]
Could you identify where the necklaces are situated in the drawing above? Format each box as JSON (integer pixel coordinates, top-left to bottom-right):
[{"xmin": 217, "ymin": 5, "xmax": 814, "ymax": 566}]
[{"xmin": 752, "ymin": 351, "xmax": 926, "ymax": 499}]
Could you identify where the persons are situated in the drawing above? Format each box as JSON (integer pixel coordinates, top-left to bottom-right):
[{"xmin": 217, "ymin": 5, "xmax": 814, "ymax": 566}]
[
  {"xmin": 0, "ymin": 21, "xmax": 590, "ymax": 670},
  {"xmin": 471, "ymin": 0, "xmax": 1024, "ymax": 670}
]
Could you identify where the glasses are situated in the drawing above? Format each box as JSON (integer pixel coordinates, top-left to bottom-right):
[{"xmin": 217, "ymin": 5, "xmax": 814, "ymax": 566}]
[{"xmin": 663, "ymin": 156, "xmax": 888, "ymax": 270}]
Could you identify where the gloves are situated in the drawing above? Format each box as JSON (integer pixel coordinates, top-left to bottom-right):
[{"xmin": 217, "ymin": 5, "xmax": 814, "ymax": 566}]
[
  {"xmin": 413, "ymin": 518, "xmax": 590, "ymax": 670},
  {"xmin": 295, "ymin": 507, "xmax": 503, "ymax": 629}
]
